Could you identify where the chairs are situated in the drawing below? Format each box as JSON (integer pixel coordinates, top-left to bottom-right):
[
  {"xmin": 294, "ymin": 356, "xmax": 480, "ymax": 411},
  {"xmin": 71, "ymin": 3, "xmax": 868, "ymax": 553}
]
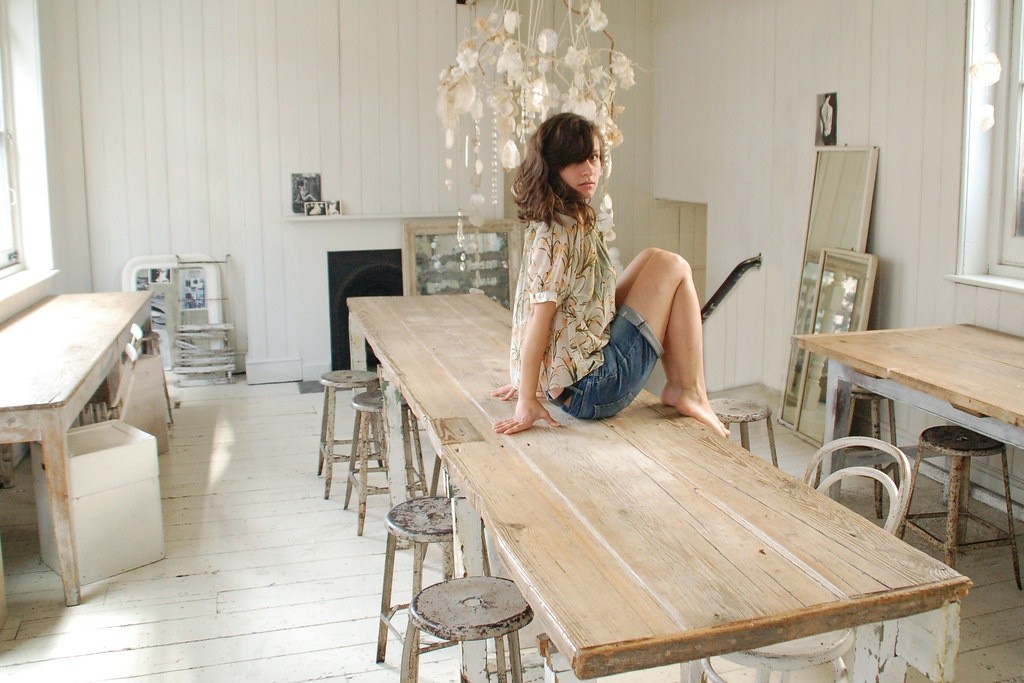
[{"xmin": 702, "ymin": 436, "xmax": 912, "ymax": 683}]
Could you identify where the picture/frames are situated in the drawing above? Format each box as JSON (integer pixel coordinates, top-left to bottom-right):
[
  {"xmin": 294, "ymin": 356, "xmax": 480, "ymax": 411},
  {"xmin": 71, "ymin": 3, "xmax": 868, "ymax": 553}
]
[
  {"xmin": 325, "ymin": 200, "xmax": 342, "ymax": 216},
  {"xmin": 400, "ymin": 218, "xmax": 521, "ymax": 311},
  {"xmin": 304, "ymin": 202, "xmax": 326, "ymax": 216}
]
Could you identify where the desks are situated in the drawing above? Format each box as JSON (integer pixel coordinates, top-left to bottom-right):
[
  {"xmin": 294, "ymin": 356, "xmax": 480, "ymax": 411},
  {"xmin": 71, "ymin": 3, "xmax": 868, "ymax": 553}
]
[
  {"xmin": 0, "ymin": 289, "xmax": 174, "ymax": 606},
  {"xmin": 346, "ymin": 296, "xmax": 975, "ymax": 683},
  {"xmin": 795, "ymin": 324, "xmax": 1024, "ymax": 592}
]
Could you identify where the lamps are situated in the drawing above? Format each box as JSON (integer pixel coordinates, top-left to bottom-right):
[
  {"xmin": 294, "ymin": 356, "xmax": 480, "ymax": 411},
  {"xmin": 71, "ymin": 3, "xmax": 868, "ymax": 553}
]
[{"xmin": 438, "ymin": 0, "xmax": 635, "ymax": 271}]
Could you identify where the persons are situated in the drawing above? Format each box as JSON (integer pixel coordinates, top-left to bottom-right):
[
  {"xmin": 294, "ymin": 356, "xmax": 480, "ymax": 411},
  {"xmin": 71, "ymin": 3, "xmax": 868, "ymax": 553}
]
[
  {"xmin": 308, "ymin": 204, "xmax": 321, "ymax": 215},
  {"xmin": 186, "ymin": 279, "xmax": 204, "ymax": 307},
  {"xmin": 156, "ymin": 270, "xmax": 168, "ymax": 282},
  {"xmin": 820, "ymin": 94, "xmax": 833, "ymax": 145},
  {"xmin": 490, "ymin": 113, "xmax": 731, "ymax": 438},
  {"xmin": 295, "ymin": 186, "xmax": 316, "ymax": 200},
  {"xmin": 328, "ymin": 203, "xmax": 338, "ymax": 215}
]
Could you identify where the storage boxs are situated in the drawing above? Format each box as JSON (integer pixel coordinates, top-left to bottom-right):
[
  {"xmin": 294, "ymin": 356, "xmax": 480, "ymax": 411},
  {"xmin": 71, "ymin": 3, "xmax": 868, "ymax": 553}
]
[
  {"xmin": 30, "ymin": 420, "xmax": 164, "ymax": 586},
  {"xmin": 107, "ymin": 354, "xmax": 169, "ymax": 455}
]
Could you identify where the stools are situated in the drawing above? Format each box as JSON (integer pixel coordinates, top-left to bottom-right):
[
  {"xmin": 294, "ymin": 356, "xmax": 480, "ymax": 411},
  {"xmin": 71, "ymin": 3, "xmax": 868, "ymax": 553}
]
[
  {"xmin": 894, "ymin": 424, "xmax": 1022, "ymax": 591},
  {"xmin": 317, "ymin": 369, "xmax": 383, "ymax": 500},
  {"xmin": 376, "ymin": 496, "xmax": 493, "ymax": 683},
  {"xmin": 343, "ymin": 390, "xmax": 429, "ymax": 536},
  {"xmin": 140, "ymin": 330, "xmax": 174, "ymax": 424},
  {"xmin": 842, "ymin": 386, "xmax": 899, "ymax": 518},
  {"xmin": 399, "ymin": 575, "xmax": 534, "ymax": 683},
  {"xmin": 709, "ymin": 399, "xmax": 779, "ymax": 467}
]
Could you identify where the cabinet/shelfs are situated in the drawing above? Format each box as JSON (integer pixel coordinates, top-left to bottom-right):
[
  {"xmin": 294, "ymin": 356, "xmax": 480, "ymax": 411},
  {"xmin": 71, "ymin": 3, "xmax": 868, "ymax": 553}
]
[{"xmin": 122, "ymin": 255, "xmax": 235, "ymax": 387}]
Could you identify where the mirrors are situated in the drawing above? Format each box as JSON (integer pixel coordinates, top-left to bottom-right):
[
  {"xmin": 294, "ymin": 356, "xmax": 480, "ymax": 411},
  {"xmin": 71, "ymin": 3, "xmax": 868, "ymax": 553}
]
[
  {"xmin": 778, "ymin": 146, "xmax": 879, "ymax": 431},
  {"xmin": 792, "ymin": 246, "xmax": 878, "ymax": 449}
]
